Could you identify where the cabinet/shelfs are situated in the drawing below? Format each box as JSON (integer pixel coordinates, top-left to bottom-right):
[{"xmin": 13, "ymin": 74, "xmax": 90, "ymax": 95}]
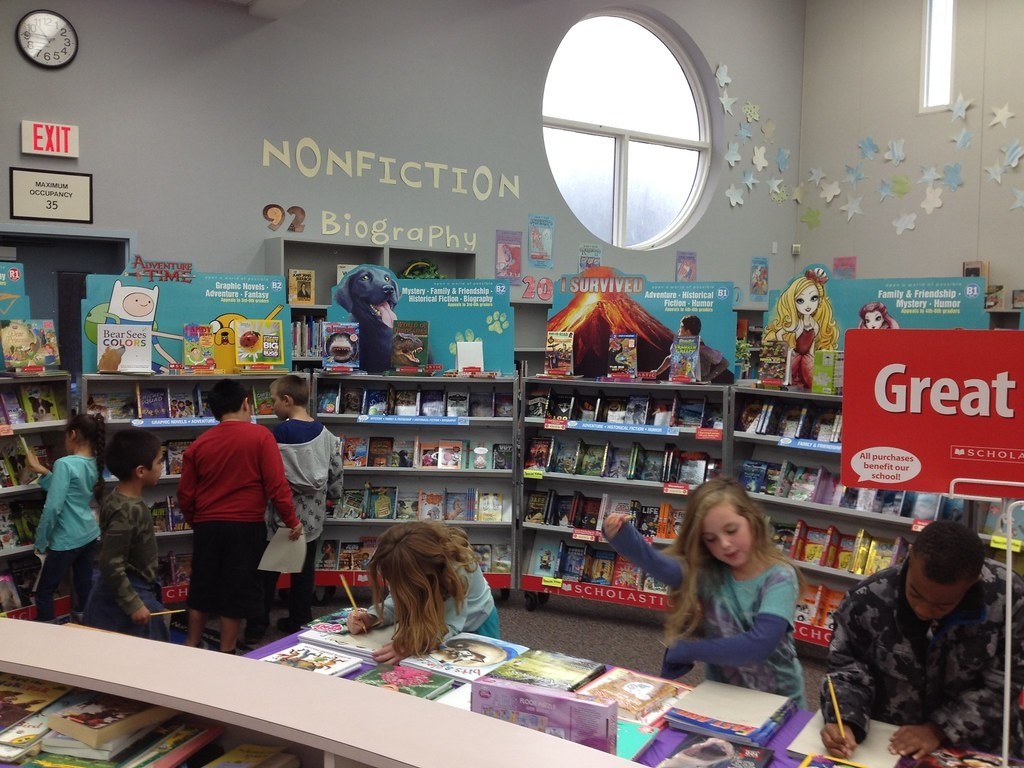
[
  {"xmin": 311, "ymin": 373, "xmax": 519, "ymax": 602},
  {"xmin": 0, "ymin": 375, "xmax": 72, "ymax": 622},
  {"xmin": 517, "ymin": 374, "xmax": 739, "ymax": 612},
  {"xmin": 77, "ymin": 373, "xmax": 311, "ymax": 604},
  {"xmin": 729, "ymin": 381, "xmax": 1024, "ymax": 712}
]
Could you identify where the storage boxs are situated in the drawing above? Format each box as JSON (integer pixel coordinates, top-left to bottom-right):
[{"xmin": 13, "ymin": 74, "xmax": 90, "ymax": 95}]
[
  {"xmin": 471, "ymin": 674, "xmax": 618, "ymax": 756},
  {"xmin": 812, "ymin": 350, "xmax": 844, "ymax": 396},
  {"xmin": 321, "ymin": 321, "xmax": 360, "ymax": 370}
]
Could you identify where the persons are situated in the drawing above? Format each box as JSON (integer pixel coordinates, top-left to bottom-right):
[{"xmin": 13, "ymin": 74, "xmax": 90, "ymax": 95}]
[
  {"xmin": 602, "ymin": 480, "xmax": 808, "ymax": 709},
  {"xmin": 447, "ymin": 498, "xmax": 465, "ymax": 520},
  {"xmin": 177, "ymin": 379, "xmax": 303, "ymax": 656},
  {"xmin": 650, "ymin": 315, "xmax": 730, "ymax": 382},
  {"xmin": 348, "ymin": 521, "xmax": 502, "ymax": 667},
  {"xmin": 319, "ymin": 539, "xmax": 337, "ymax": 569},
  {"xmin": 26, "ymin": 413, "xmax": 105, "ymax": 624},
  {"xmin": 79, "ymin": 428, "xmax": 169, "ymax": 642},
  {"xmin": 818, "ymin": 519, "xmax": 1024, "ymax": 761},
  {"xmin": 245, "ymin": 376, "xmax": 343, "ymax": 646}
]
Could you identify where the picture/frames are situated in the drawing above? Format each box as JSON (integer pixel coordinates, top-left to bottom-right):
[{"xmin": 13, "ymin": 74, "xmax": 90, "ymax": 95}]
[{"xmin": 9, "ymin": 166, "xmax": 93, "ymax": 224}]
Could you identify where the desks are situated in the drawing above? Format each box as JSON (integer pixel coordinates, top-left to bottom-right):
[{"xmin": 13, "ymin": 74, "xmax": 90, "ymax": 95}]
[{"xmin": 241, "ymin": 630, "xmax": 1024, "ymax": 768}]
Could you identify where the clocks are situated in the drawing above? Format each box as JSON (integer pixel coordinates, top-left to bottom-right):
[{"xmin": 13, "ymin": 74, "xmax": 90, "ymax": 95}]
[{"xmin": 14, "ymin": 9, "xmax": 80, "ymax": 71}]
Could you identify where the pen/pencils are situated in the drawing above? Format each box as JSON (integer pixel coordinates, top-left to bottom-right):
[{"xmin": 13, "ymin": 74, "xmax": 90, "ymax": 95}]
[
  {"xmin": 150, "ymin": 609, "xmax": 186, "ymax": 616},
  {"xmin": 339, "ymin": 573, "xmax": 367, "ymax": 633},
  {"xmin": 825, "ymin": 673, "xmax": 851, "ymax": 759},
  {"xmin": 608, "ymin": 514, "xmax": 634, "ymax": 524}
]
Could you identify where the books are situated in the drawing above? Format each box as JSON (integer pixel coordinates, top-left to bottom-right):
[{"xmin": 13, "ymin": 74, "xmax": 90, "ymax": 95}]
[
  {"xmin": 288, "ymin": 269, "xmax": 315, "ymax": 306},
  {"xmin": 0, "ymin": 320, "xmax": 1024, "ymax": 768},
  {"xmin": 962, "ymin": 260, "xmax": 990, "ymax": 293}
]
